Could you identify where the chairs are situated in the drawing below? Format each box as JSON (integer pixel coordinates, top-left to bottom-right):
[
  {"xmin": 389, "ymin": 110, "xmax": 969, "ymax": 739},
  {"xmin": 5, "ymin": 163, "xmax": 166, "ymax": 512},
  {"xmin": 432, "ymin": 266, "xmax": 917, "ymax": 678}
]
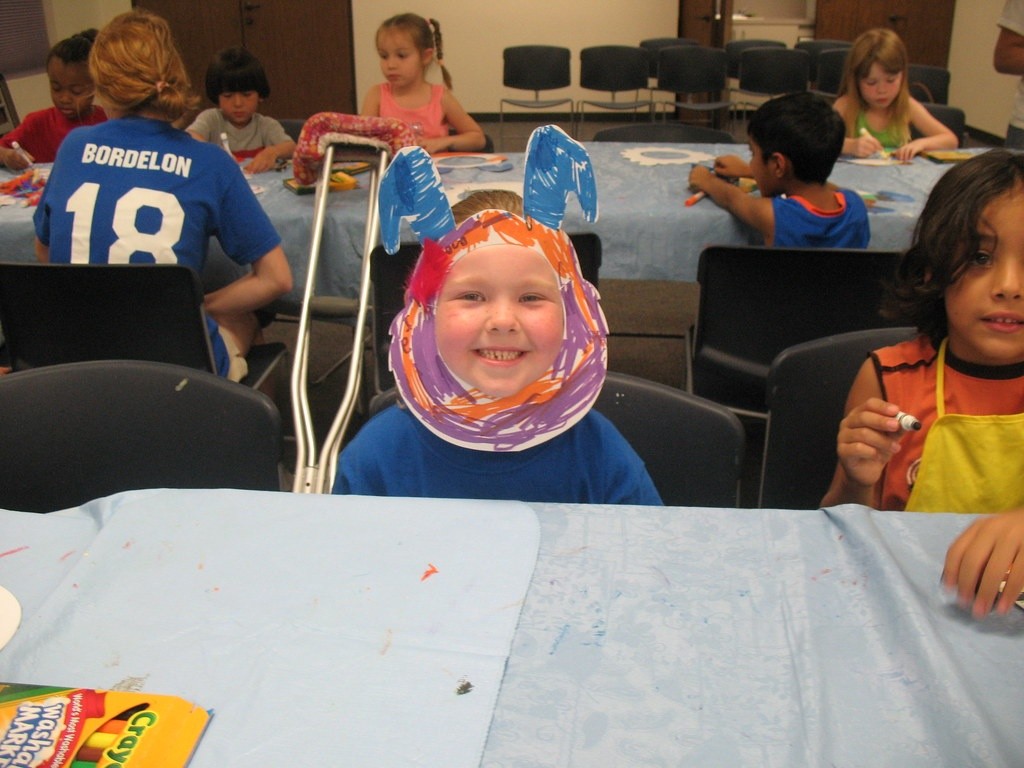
[
  {"xmin": 499, "ymin": 44, "xmax": 574, "ymax": 148},
  {"xmin": 907, "ymin": 63, "xmax": 951, "ymax": 105},
  {"xmin": 912, "ymin": 102, "xmax": 967, "ymax": 149},
  {"xmin": 0, "ymin": 261, "xmax": 296, "ymax": 445},
  {"xmin": 817, "ymin": 47, "xmax": 854, "ymax": 94},
  {"xmin": 638, "ymin": 38, "xmax": 701, "ymax": 121},
  {"xmin": 366, "ymin": 374, "xmax": 752, "ymax": 503},
  {"xmin": 364, "ymin": 231, "xmax": 602, "ymax": 399},
  {"xmin": 792, "ymin": 39, "xmax": 854, "ymax": 90},
  {"xmin": 577, "ymin": 46, "xmax": 657, "ymax": 139},
  {"xmin": 592, "ymin": 123, "xmax": 734, "ymax": 143},
  {"xmin": 0, "ymin": 358, "xmax": 283, "ymax": 511},
  {"xmin": 743, "ymin": 47, "xmax": 813, "ymax": 136},
  {"xmin": 658, "ymin": 45, "xmax": 737, "ymax": 131},
  {"xmin": 686, "ymin": 245, "xmax": 917, "ymax": 412},
  {"xmin": 278, "ymin": 118, "xmax": 306, "ymax": 143},
  {"xmin": 759, "ymin": 325, "xmax": 922, "ymax": 508},
  {"xmin": 726, "ymin": 39, "xmax": 790, "ymax": 127}
]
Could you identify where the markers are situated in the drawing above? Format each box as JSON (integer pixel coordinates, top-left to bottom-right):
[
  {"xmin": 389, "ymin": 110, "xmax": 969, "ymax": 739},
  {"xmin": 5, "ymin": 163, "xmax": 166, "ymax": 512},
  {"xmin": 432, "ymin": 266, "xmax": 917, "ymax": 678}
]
[
  {"xmin": 859, "ymin": 127, "xmax": 888, "ymax": 160},
  {"xmin": 220, "ymin": 132, "xmax": 232, "ymax": 156},
  {"xmin": 11, "ymin": 141, "xmax": 35, "ymax": 170},
  {"xmin": 895, "ymin": 411, "xmax": 922, "ymax": 431},
  {"xmin": 684, "ymin": 191, "xmax": 706, "ymax": 206}
]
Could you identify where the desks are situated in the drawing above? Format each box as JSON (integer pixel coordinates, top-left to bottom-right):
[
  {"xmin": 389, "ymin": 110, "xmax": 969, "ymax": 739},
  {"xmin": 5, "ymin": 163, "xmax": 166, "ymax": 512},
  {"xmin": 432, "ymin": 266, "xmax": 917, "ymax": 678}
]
[
  {"xmin": 0, "ymin": 491, "xmax": 1024, "ymax": 768},
  {"xmin": 0, "ymin": 142, "xmax": 1024, "ymax": 284}
]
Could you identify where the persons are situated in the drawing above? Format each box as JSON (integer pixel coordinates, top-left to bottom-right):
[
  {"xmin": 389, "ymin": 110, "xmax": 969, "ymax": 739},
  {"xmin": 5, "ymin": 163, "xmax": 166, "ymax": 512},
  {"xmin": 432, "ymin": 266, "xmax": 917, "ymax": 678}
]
[
  {"xmin": 359, "ymin": 13, "xmax": 486, "ymax": 156},
  {"xmin": 831, "ymin": 29, "xmax": 959, "ymax": 161},
  {"xmin": 33, "ymin": 11, "xmax": 293, "ymax": 452},
  {"xmin": 0, "ymin": 28, "xmax": 108, "ymax": 171},
  {"xmin": 818, "ymin": 149, "xmax": 1024, "ymax": 620},
  {"xmin": 690, "ymin": 92, "xmax": 871, "ymax": 252},
  {"xmin": 184, "ymin": 48, "xmax": 299, "ymax": 175},
  {"xmin": 330, "ymin": 190, "xmax": 665, "ymax": 506}
]
